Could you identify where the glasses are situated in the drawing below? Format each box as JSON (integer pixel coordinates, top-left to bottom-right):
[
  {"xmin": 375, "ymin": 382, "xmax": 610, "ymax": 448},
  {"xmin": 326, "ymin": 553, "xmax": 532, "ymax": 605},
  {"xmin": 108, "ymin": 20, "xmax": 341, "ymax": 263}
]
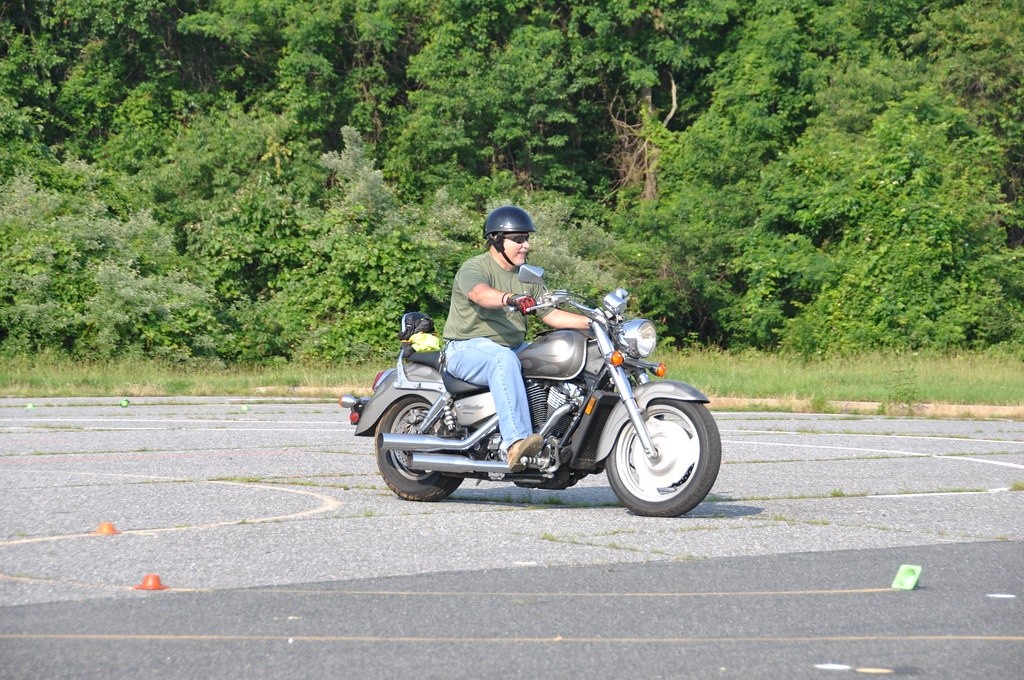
[{"xmin": 503, "ymin": 235, "xmax": 529, "ymax": 244}]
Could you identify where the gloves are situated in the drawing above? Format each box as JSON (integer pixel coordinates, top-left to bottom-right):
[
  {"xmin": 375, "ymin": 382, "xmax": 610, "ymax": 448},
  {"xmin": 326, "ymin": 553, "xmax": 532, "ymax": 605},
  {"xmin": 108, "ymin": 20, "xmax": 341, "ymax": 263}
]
[{"xmin": 506, "ymin": 294, "xmax": 537, "ymax": 315}]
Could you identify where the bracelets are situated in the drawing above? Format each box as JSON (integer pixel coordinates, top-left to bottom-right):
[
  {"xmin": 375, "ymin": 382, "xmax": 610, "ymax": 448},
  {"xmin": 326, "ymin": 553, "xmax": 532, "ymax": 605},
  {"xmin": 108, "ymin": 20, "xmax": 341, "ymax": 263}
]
[{"xmin": 501, "ymin": 293, "xmax": 509, "ymax": 307}]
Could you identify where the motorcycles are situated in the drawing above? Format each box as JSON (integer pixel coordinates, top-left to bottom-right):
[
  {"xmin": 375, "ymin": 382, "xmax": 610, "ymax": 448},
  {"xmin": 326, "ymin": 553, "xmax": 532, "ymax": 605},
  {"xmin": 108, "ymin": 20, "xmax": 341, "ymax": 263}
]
[{"xmin": 338, "ymin": 264, "xmax": 722, "ymax": 518}]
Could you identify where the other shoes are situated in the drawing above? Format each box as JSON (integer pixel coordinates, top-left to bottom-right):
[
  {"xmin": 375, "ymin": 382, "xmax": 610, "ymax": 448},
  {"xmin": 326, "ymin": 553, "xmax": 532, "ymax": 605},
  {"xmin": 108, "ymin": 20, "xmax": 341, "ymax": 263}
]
[{"xmin": 507, "ymin": 434, "xmax": 544, "ymax": 472}]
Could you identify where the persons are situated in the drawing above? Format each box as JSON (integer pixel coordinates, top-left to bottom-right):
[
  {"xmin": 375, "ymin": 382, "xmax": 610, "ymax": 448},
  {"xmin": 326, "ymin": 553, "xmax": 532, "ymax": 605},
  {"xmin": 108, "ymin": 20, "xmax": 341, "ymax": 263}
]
[{"xmin": 443, "ymin": 207, "xmax": 589, "ymax": 472}]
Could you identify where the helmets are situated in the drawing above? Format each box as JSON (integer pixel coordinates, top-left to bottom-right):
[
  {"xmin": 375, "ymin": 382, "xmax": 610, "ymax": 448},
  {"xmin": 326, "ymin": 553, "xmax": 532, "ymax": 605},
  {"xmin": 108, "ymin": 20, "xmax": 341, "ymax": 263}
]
[{"xmin": 483, "ymin": 206, "xmax": 537, "ymax": 239}]
